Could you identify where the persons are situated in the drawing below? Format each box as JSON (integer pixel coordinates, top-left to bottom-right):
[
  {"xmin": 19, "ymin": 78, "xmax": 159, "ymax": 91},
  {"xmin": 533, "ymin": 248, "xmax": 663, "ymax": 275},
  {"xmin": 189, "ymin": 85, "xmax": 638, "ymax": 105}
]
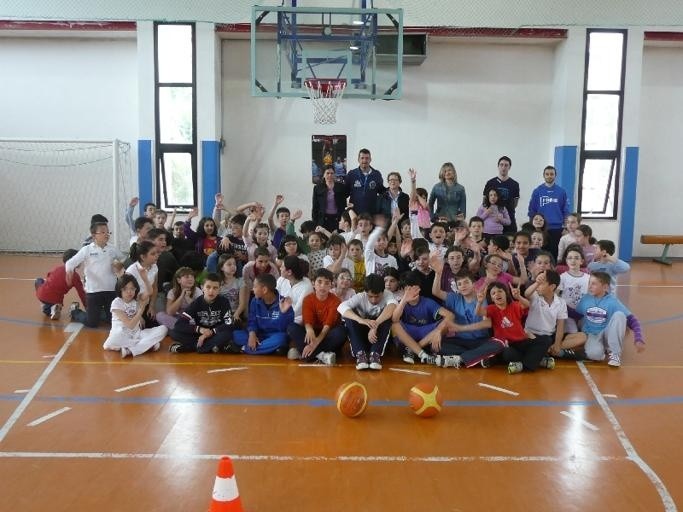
[
  {"xmin": 312, "ymin": 142, "xmax": 346, "ymax": 183},
  {"xmin": 335, "ymin": 272, "xmax": 399, "ymax": 371},
  {"xmin": 311, "ymin": 164, "xmax": 347, "ymax": 234},
  {"xmin": 286, "ymin": 267, "xmax": 348, "ymax": 365},
  {"xmin": 100, "ymin": 273, "xmax": 168, "ymax": 359},
  {"xmin": 481, "ymin": 155, "xmax": 521, "ymax": 239},
  {"xmin": 168, "ymin": 272, "xmax": 236, "ymax": 354},
  {"xmin": 526, "ymin": 165, "xmax": 570, "ymax": 264},
  {"xmin": 343, "ymin": 148, "xmax": 387, "ymax": 223},
  {"xmin": 427, "ymin": 260, "xmax": 493, "ymax": 366},
  {"xmin": 427, "ymin": 161, "xmax": 467, "ymax": 232},
  {"xmin": 81, "ymin": 166, "xmax": 632, "ymax": 327},
  {"xmin": 523, "ymin": 266, "xmax": 587, "ymax": 361},
  {"xmin": 64, "ymin": 222, "xmax": 125, "ymax": 327},
  {"xmin": 33, "ymin": 248, "xmax": 87, "ymax": 321},
  {"xmin": 231, "ymin": 273, "xmax": 295, "ymax": 355},
  {"xmin": 571, "ymin": 272, "xmax": 647, "ymax": 367},
  {"xmin": 475, "ymin": 278, "xmax": 555, "ymax": 372},
  {"xmin": 390, "ymin": 271, "xmax": 454, "ymax": 366}
]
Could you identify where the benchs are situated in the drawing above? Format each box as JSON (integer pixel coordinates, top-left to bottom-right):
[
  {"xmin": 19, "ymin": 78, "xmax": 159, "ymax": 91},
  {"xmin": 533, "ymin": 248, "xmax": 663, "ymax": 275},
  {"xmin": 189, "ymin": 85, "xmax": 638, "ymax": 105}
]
[{"xmin": 640, "ymin": 234, "xmax": 683, "ymax": 265}]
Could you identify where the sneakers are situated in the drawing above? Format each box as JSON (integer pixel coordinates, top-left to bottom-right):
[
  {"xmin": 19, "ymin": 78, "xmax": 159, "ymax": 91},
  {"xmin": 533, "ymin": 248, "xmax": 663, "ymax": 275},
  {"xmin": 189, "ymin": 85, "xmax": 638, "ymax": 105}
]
[
  {"xmin": 275, "ymin": 347, "xmax": 285, "ymax": 354},
  {"xmin": 119, "ymin": 347, "xmax": 131, "ymax": 359},
  {"xmin": 168, "ymin": 342, "xmax": 184, "ymax": 353},
  {"xmin": 162, "ymin": 283, "xmax": 172, "ymax": 298},
  {"xmin": 402, "ymin": 347, "xmax": 415, "ymax": 364},
  {"xmin": 288, "ymin": 347, "xmax": 303, "ymax": 360},
  {"xmin": 223, "ymin": 343, "xmax": 233, "ymax": 352},
  {"xmin": 152, "ymin": 342, "xmax": 161, "ymax": 352},
  {"xmin": 50, "ymin": 302, "xmax": 79, "ymax": 322},
  {"xmin": 480, "ymin": 359, "xmax": 497, "ymax": 368},
  {"xmin": 608, "ymin": 353, "xmax": 621, "ymax": 367},
  {"xmin": 368, "ymin": 352, "xmax": 383, "ymax": 370},
  {"xmin": 508, "ymin": 361, "xmax": 524, "ymax": 375},
  {"xmin": 355, "ymin": 350, "xmax": 369, "ymax": 370},
  {"xmin": 539, "ymin": 357, "xmax": 555, "ymax": 370},
  {"xmin": 420, "ymin": 354, "xmax": 461, "ymax": 368},
  {"xmin": 240, "ymin": 345, "xmax": 246, "ymax": 354},
  {"xmin": 321, "ymin": 352, "xmax": 337, "ymax": 364},
  {"xmin": 562, "ymin": 349, "xmax": 586, "ymax": 360}
]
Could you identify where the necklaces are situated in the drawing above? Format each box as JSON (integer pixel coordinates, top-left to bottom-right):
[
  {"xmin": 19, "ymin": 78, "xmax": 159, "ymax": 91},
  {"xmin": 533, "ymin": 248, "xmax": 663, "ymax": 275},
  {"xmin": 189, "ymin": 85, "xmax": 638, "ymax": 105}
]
[{"xmin": 592, "ymin": 297, "xmax": 604, "ymax": 309}]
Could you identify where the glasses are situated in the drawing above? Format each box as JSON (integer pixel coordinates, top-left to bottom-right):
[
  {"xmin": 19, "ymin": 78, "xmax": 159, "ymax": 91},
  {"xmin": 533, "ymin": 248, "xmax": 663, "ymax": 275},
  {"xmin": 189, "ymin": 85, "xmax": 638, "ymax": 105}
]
[{"xmin": 94, "ymin": 231, "xmax": 110, "ymax": 237}]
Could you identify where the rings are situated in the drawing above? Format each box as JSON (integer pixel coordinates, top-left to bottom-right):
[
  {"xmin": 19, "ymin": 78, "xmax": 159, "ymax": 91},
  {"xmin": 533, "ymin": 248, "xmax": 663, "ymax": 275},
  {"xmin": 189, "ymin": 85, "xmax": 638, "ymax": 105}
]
[{"xmin": 367, "ymin": 321, "xmax": 370, "ymax": 324}]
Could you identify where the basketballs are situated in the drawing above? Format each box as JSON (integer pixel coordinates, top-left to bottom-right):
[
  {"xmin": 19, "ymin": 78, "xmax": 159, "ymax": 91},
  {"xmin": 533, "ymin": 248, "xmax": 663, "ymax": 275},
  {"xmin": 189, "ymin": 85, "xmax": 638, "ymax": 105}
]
[
  {"xmin": 335, "ymin": 381, "xmax": 369, "ymax": 418},
  {"xmin": 408, "ymin": 383, "xmax": 443, "ymax": 418}
]
